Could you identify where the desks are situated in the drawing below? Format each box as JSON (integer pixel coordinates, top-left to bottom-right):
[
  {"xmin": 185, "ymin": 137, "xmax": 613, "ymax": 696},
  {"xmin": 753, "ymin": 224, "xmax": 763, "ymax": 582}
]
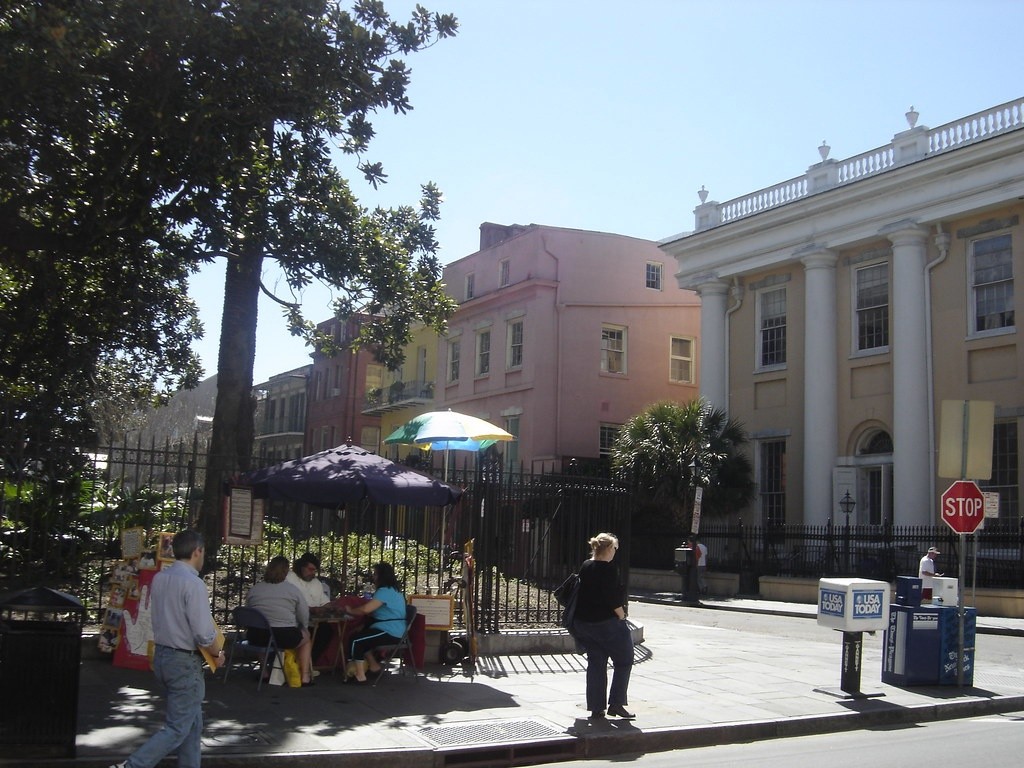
[{"xmin": 305, "ymin": 613, "xmax": 352, "ymax": 682}]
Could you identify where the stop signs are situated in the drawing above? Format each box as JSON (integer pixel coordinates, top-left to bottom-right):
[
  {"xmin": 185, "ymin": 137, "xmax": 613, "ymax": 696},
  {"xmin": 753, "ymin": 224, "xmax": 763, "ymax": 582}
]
[{"xmin": 941, "ymin": 481, "xmax": 986, "ymax": 534}]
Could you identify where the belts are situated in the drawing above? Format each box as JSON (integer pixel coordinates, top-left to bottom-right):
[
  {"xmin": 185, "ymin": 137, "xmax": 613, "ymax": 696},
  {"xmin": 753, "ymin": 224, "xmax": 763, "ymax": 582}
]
[{"xmin": 156, "ymin": 644, "xmax": 198, "ymax": 654}]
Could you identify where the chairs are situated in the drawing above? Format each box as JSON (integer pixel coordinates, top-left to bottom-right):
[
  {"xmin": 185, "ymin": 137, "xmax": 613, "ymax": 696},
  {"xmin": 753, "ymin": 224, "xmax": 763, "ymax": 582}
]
[
  {"xmin": 222, "ymin": 606, "xmax": 288, "ymax": 693},
  {"xmin": 367, "ymin": 604, "xmax": 422, "ymax": 687}
]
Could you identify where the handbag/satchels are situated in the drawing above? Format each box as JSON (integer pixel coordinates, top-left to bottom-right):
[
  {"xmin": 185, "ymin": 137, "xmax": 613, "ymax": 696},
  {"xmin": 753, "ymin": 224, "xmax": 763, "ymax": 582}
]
[
  {"xmin": 269, "ymin": 651, "xmax": 284, "ymax": 686},
  {"xmin": 555, "ymin": 573, "xmax": 579, "ymax": 607},
  {"xmin": 283, "ymin": 649, "xmax": 301, "ymax": 687}
]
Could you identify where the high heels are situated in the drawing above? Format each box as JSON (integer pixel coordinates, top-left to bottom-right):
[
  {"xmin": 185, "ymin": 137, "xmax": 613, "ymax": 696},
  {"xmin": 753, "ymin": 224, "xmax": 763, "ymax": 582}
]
[
  {"xmin": 593, "ymin": 710, "xmax": 605, "ymax": 718},
  {"xmin": 607, "ymin": 705, "xmax": 636, "ymax": 718}
]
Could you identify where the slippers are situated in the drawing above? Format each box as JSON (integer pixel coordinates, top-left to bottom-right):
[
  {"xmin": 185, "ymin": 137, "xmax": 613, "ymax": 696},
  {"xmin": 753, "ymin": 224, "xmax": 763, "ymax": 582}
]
[
  {"xmin": 365, "ymin": 670, "xmax": 382, "ymax": 678},
  {"xmin": 344, "ymin": 676, "xmax": 368, "ymax": 685}
]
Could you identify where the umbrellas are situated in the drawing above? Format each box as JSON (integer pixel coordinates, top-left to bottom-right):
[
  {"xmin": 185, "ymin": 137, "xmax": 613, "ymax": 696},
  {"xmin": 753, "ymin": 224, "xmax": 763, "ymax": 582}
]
[
  {"xmin": 230, "ymin": 436, "xmax": 460, "ymax": 586},
  {"xmin": 382, "ymin": 408, "xmax": 516, "ymax": 592}
]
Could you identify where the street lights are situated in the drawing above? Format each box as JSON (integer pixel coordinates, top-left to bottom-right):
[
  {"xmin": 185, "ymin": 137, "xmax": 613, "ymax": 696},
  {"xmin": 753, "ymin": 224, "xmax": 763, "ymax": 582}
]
[
  {"xmin": 683, "ymin": 454, "xmax": 704, "ymax": 600},
  {"xmin": 838, "ymin": 490, "xmax": 856, "ymax": 575}
]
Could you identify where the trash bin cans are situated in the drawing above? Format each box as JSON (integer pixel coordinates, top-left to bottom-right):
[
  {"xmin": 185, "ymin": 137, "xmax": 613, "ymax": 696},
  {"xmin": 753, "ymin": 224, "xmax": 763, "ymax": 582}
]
[{"xmin": 0, "ymin": 587, "xmax": 87, "ymax": 761}]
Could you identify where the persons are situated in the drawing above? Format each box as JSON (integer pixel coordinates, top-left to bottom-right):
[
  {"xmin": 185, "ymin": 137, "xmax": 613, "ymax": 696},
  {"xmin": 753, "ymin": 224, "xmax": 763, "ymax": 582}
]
[
  {"xmin": 245, "ymin": 556, "xmax": 315, "ymax": 687},
  {"xmin": 578, "ymin": 532, "xmax": 636, "ymax": 719},
  {"xmin": 109, "ymin": 530, "xmax": 225, "ymax": 768},
  {"xmin": 345, "ymin": 565, "xmax": 407, "ymax": 684},
  {"xmin": 686, "ymin": 535, "xmax": 708, "ymax": 595},
  {"xmin": 285, "ymin": 551, "xmax": 330, "ymax": 676},
  {"xmin": 918, "ymin": 547, "xmax": 940, "ymax": 590}
]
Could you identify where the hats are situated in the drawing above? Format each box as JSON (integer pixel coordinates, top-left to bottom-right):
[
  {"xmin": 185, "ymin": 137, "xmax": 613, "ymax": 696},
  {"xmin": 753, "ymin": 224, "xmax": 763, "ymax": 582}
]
[{"xmin": 928, "ymin": 547, "xmax": 940, "ymax": 554}]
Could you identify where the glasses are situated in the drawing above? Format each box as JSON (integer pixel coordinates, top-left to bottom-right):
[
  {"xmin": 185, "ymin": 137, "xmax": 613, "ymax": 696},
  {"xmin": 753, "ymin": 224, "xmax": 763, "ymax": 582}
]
[{"xmin": 305, "ymin": 566, "xmax": 317, "ymax": 572}]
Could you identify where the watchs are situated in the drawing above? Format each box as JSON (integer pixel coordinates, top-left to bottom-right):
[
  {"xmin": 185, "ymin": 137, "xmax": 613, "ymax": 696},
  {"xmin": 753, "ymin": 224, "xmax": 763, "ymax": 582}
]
[{"xmin": 214, "ymin": 650, "xmax": 222, "ymax": 658}]
